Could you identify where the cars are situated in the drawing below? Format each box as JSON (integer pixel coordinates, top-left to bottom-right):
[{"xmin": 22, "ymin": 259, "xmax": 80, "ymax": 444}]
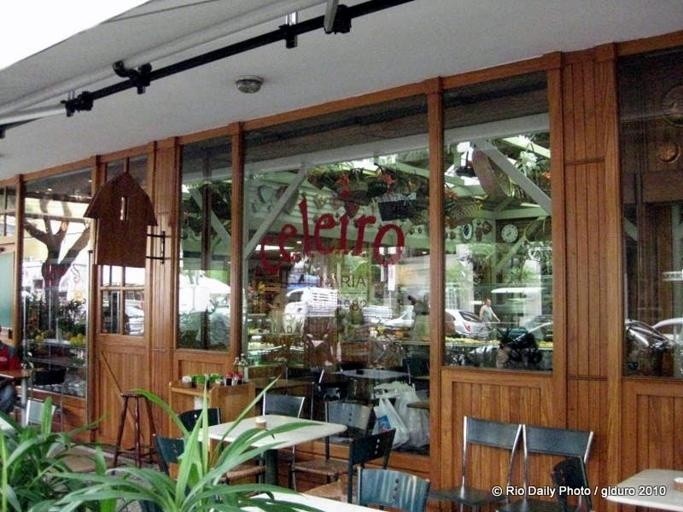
[
  {"xmin": 384, "ymin": 308, "xmax": 488, "ymax": 340},
  {"xmin": 469, "ymin": 317, "xmax": 678, "ymax": 377}
]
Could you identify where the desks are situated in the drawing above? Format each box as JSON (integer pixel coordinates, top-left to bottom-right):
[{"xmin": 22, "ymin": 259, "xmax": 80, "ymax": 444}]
[
  {"xmin": 198, "ymin": 411, "xmax": 346, "ymax": 486},
  {"xmin": 1, "ymin": 367, "xmax": 36, "ymax": 429},
  {"xmin": 190, "ymin": 492, "xmax": 393, "ymax": 512},
  {"xmin": 604, "ymin": 467, "xmax": 683, "ymax": 512}
]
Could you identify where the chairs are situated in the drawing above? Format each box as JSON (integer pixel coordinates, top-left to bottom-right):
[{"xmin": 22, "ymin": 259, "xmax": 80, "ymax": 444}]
[
  {"xmin": 494, "ymin": 424, "xmax": 596, "ymax": 512},
  {"xmin": 25, "ymin": 368, "xmax": 67, "ymax": 433},
  {"xmin": 263, "ymin": 395, "xmax": 303, "ymax": 493},
  {"xmin": 291, "ymin": 379, "xmax": 372, "ymax": 495},
  {"xmin": 174, "ymin": 408, "xmax": 227, "ymax": 485},
  {"xmin": 358, "ymin": 464, "xmax": 430, "ymax": 512},
  {"xmin": 427, "ymin": 415, "xmax": 523, "ymax": 512},
  {"xmin": 151, "ymin": 438, "xmax": 192, "ymax": 490},
  {"xmin": 303, "ymin": 428, "xmax": 396, "ymax": 504}
]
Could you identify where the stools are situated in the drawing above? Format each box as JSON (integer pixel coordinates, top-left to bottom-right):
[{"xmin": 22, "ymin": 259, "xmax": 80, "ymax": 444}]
[{"xmin": 111, "ymin": 392, "xmax": 167, "ymax": 478}]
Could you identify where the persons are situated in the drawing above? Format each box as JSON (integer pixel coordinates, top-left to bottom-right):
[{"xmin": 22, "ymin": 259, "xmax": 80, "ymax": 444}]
[
  {"xmin": 0, "ymin": 341, "xmax": 20, "ymax": 415},
  {"xmin": 477, "ymin": 298, "xmax": 500, "ymax": 324}
]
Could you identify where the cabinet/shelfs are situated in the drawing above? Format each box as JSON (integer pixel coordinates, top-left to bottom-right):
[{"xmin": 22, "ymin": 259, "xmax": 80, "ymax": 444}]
[{"xmin": 168, "ymin": 377, "xmax": 258, "ymax": 488}]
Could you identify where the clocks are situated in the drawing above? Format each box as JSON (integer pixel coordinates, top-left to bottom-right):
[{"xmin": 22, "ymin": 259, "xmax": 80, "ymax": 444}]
[{"xmin": 501, "ymin": 225, "xmax": 519, "ymax": 244}]
